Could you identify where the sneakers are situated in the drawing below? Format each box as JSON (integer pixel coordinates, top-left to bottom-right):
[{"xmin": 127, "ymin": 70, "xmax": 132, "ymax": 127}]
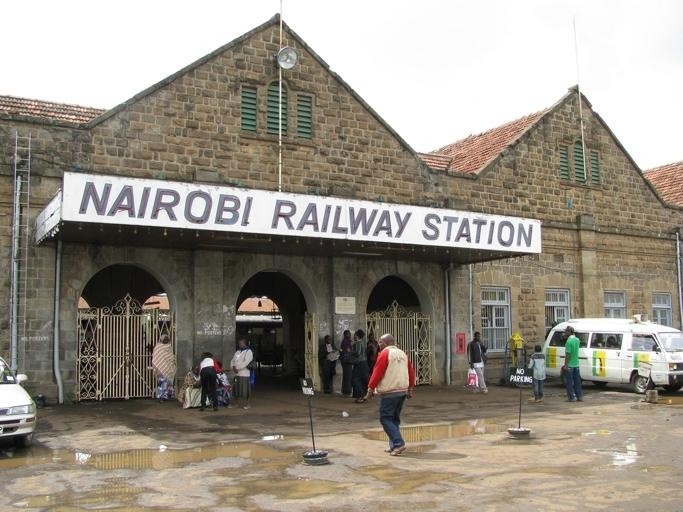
[
  {"xmin": 384, "ymin": 447, "xmax": 392, "ymax": 452},
  {"xmin": 390, "ymin": 445, "xmax": 406, "ymax": 456}
]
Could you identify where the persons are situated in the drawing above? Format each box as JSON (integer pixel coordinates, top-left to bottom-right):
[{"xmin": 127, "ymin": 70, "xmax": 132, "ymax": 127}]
[
  {"xmin": 318, "ymin": 335, "xmax": 340, "ymax": 394},
  {"xmin": 467, "ymin": 332, "xmax": 489, "ymax": 394},
  {"xmin": 562, "ymin": 325, "xmax": 584, "ymax": 403},
  {"xmin": 199, "ymin": 352, "xmax": 224, "ymax": 412},
  {"xmin": 362, "ymin": 333, "xmax": 417, "ymax": 457},
  {"xmin": 229, "ymin": 338, "xmax": 254, "ymax": 410},
  {"xmin": 526, "ymin": 345, "xmax": 547, "ymax": 403},
  {"xmin": 365, "ymin": 333, "xmax": 381, "ymax": 376},
  {"xmin": 340, "ymin": 330, "xmax": 354, "ymax": 398},
  {"xmin": 338, "ymin": 328, "xmax": 370, "ymax": 404}
]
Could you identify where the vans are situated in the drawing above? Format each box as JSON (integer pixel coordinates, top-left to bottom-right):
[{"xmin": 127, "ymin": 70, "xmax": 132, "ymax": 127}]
[{"xmin": 542, "ymin": 312, "xmax": 683, "ymax": 393}]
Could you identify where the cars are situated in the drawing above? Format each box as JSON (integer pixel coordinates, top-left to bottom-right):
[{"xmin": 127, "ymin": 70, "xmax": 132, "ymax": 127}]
[{"xmin": 0, "ymin": 356, "xmax": 37, "ymax": 448}]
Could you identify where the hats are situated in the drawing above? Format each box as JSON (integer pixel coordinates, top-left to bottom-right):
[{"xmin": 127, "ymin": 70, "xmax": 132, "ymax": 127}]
[{"xmin": 563, "ymin": 325, "xmax": 574, "ymax": 333}]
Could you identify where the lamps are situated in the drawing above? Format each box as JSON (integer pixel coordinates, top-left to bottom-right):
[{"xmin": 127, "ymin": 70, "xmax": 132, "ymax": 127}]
[{"xmin": 274, "ymin": 46, "xmax": 299, "ymax": 70}]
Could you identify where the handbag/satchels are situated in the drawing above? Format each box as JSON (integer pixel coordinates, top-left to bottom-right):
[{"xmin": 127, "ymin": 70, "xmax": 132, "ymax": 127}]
[
  {"xmin": 464, "ymin": 368, "xmax": 479, "ymax": 389},
  {"xmin": 483, "ymin": 355, "xmax": 487, "ymax": 363},
  {"xmin": 244, "ymin": 348, "xmax": 258, "ymax": 370}
]
